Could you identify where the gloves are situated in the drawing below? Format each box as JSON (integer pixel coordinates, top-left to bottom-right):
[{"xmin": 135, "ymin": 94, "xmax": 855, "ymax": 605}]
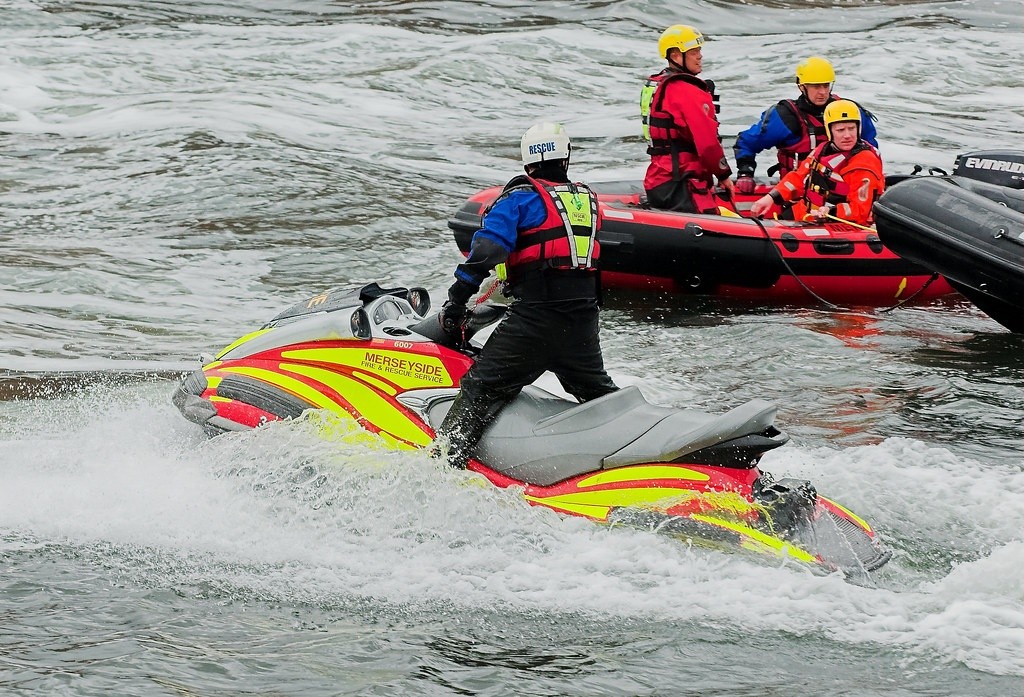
[
  {"xmin": 736, "ymin": 176, "xmax": 756, "ymax": 194},
  {"xmin": 438, "ymin": 299, "xmax": 466, "ymax": 332}
]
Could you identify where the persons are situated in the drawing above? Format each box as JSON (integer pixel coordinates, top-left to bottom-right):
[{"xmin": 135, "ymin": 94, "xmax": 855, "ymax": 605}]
[
  {"xmin": 430, "ymin": 124, "xmax": 620, "ymax": 470},
  {"xmin": 750, "ymin": 101, "xmax": 884, "ymax": 224},
  {"xmin": 733, "ymin": 58, "xmax": 878, "ymax": 193},
  {"xmin": 640, "ymin": 25, "xmax": 735, "ymax": 216}
]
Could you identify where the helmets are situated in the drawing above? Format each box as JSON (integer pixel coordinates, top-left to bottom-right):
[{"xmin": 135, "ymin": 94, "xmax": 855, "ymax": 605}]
[
  {"xmin": 659, "ymin": 24, "xmax": 704, "ymax": 59},
  {"xmin": 796, "ymin": 57, "xmax": 836, "ymax": 85},
  {"xmin": 824, "ymin": 100, "xmax": 862, "ymax": 142},
  {"xmin": 520, "ymin": 122, "xmax": 573, "ymax": 167}
]
